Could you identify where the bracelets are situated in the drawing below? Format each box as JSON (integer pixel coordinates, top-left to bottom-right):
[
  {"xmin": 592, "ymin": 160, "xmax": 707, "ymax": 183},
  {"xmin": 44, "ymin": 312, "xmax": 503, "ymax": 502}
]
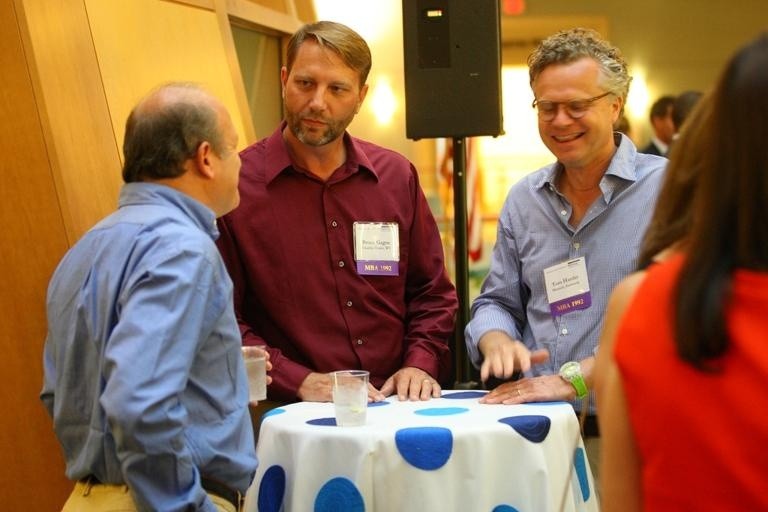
[{"xmin": 559, "ymin": 360, "xmax": 589, "ymax": 399}]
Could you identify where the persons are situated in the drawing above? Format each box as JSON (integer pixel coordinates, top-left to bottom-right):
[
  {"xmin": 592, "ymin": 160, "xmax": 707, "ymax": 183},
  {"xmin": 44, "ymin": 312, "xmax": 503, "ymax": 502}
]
[
  {"xmin": 637, "ymin": 95, "xmax": 679, "ymax": 159},
  {"xmin": 596, "ymin": 28, "xmax": 768, "ymax": 512},
  {"xmin": 672, "ymin": 88, "xmax": 703, "ymax": 133},
  {"xmin": 464, "ymin": 26, "xmax": 681, "ymax": 407},
  {"xmin": 204, "ymin": 17, "xmax": 461, "ymax": 447},
  {"xmin": 35, "ymin": 77, "xmax": 274, "ymax": 512}
]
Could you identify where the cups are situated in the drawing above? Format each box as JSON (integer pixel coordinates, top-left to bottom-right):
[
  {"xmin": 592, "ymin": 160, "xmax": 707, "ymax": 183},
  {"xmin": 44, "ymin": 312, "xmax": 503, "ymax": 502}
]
[
  {"xmin": 330, "ymin": 369, "xmax": 370, "ymax": 429},
  {"xmin": 242, "ymin": 345, "xmax": 268, "ymax": 403}
]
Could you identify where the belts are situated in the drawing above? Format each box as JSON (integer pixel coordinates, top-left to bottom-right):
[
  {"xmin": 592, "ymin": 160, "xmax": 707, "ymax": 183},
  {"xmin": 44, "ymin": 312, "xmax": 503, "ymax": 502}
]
[{"xmin": 78, "ymin": 473, "xmax": 243, "ymax": 512}]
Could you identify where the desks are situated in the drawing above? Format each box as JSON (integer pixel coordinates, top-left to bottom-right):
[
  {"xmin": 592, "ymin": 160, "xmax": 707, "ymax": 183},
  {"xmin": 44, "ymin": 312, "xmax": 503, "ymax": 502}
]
[{"xmin": 241, "ymin": 389, "xmax": 601, "ymax": 512}]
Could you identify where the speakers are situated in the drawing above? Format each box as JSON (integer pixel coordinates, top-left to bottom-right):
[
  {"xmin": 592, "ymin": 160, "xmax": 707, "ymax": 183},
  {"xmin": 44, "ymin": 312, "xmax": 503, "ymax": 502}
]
[{"xmin": 402, "ymin": 0, "xmax": 505, "ymax": 142}]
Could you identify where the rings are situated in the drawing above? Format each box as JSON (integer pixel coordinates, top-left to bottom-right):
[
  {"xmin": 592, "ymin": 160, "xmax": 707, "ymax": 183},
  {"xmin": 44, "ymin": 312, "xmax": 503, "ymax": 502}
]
[
  {"xmin": 423, "ymin": 378, "xmax": 430, "ymax": 384},
  {"xmin": 517, "ymin": 390, "xmax": 522, "ymax": 395},
  {"xmin": 430, "ymin": 379, "xmax": 437, "ymax": 385},
  {"xmin": 516, "ymin": 383, "xmax": 520, "ymax": 390}
]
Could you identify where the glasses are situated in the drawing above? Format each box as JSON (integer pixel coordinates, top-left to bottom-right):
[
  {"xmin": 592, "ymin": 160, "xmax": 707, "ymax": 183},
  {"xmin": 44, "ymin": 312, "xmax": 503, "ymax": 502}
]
[{"xmin": 532, "ymin": 92, "xmax": 612, "ymax": 121}]
